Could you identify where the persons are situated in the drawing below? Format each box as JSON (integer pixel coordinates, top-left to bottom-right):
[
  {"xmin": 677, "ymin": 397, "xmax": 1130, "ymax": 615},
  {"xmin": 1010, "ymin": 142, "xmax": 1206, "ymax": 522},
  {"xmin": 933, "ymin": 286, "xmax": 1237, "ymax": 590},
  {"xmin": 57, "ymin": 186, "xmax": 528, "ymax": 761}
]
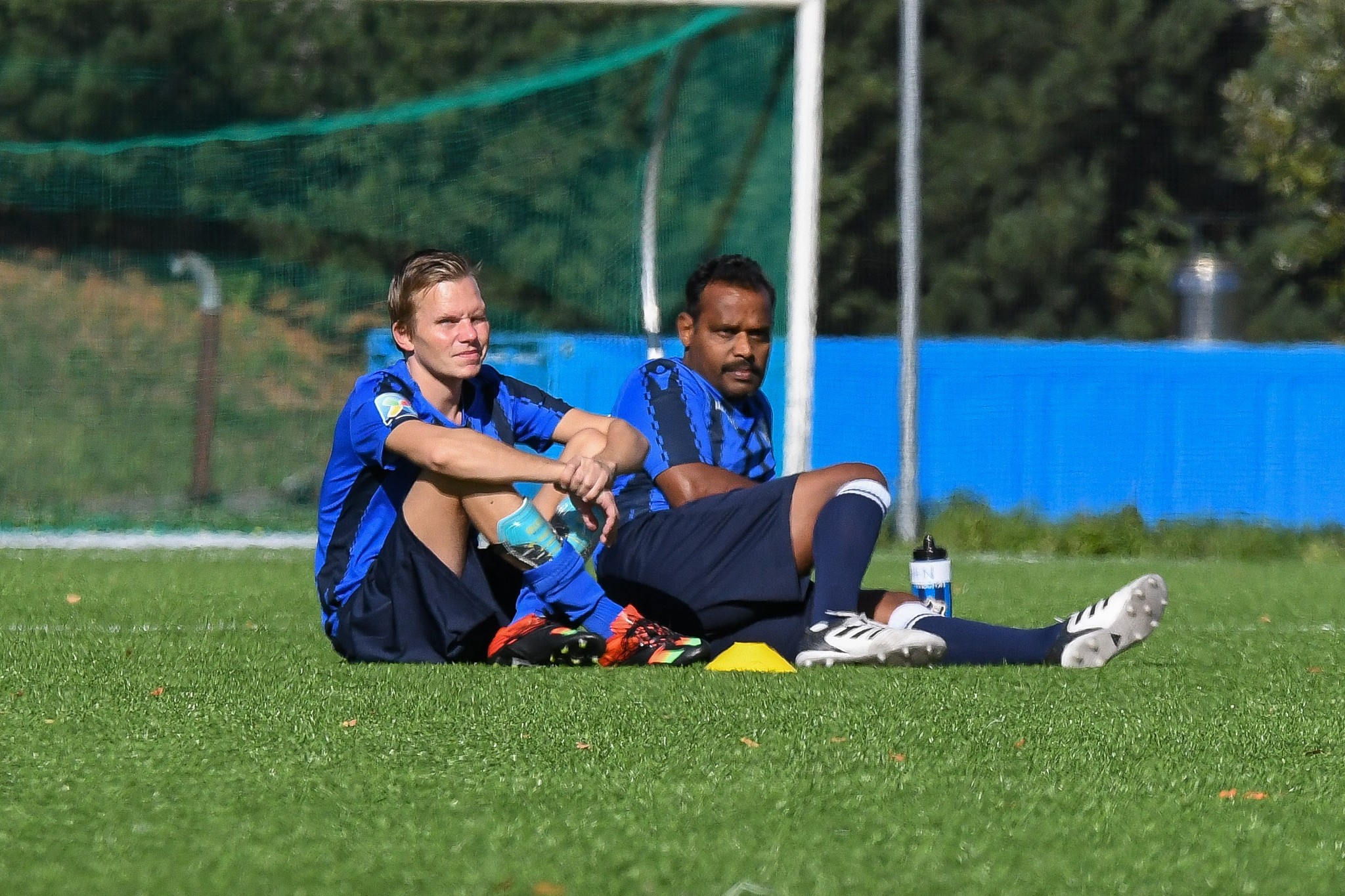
[
  {"xmin": 313, "ymin": 253, "xmax": 707, "ymax": 666},
  {"xmin": 593, "ymin": 253, "xmax": 1166, "ymax": 666}
]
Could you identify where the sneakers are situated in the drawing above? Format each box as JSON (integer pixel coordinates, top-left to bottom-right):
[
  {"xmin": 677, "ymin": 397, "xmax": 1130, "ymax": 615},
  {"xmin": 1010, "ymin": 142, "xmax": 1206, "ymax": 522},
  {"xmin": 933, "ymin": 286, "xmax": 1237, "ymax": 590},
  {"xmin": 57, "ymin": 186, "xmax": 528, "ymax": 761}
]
[
  {"xmin": 488, "ymin": 613, "xmax": 607, "ymax": 669},
  {"xmin": 1044, "ymin": 572, "xmax": 1169, "ymax": 670},
  {"xmin": 794, "ymin": 608, "xmax": 948, "ymax": 670},
  {"xmin": 601, "ymin": 603, "xmax": 711, "ymax": 667}
]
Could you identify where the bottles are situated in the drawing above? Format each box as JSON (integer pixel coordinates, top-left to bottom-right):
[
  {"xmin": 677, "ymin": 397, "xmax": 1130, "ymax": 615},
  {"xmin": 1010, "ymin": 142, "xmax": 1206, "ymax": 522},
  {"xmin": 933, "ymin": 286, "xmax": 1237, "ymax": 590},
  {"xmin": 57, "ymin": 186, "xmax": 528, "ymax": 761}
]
[{"xmin": 909, "ymin": 535, "xmax": 952, "ymax": 618}]
